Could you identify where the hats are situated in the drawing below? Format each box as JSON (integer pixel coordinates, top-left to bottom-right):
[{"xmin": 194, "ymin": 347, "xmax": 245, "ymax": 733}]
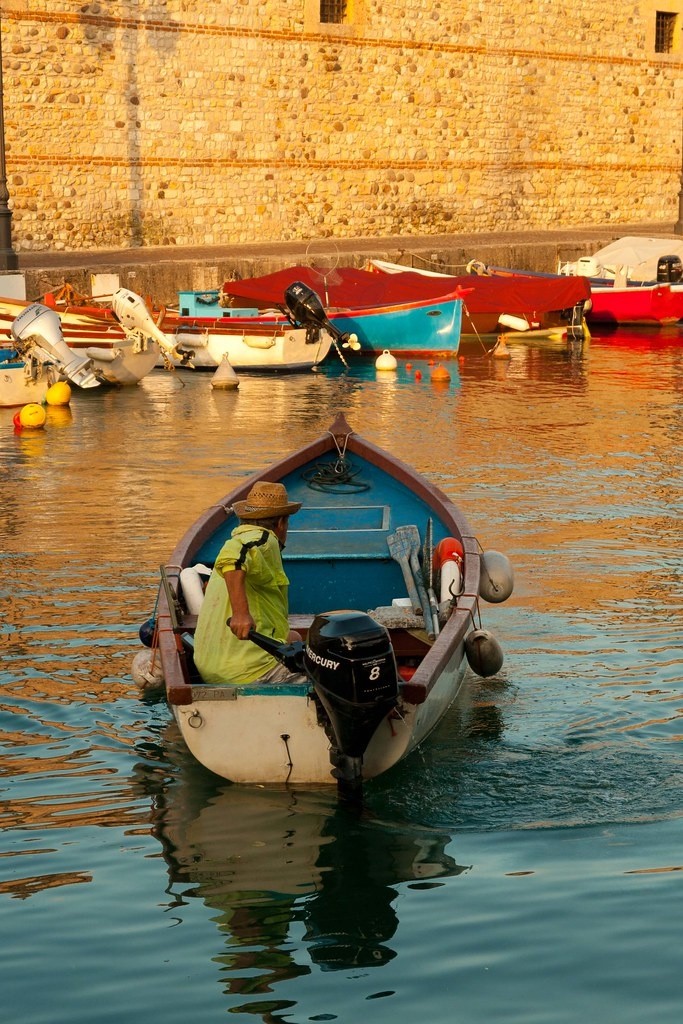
[{"xmin": 232, "ymin": 481, "xmax": 303, "ymax": 518}]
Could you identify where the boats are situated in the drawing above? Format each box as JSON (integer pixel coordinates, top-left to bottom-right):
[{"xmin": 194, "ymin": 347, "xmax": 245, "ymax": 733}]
[
  {"xmin": 132, "ymin": 412, "xmax": 516, "ymax": 792},
  {"xmin": 365, "ymin": 251, "xmax": 592, "ymax": 347},
  {"xmin": 220, "ymin": 266, "xmax": 474, "ymax": 356},
  {"xmin": 0, "ymin": 300, "xmax": 99, "ymax": 407},
  {"xmin": 140, "ymin": 286, "xmax": 337, "ymax": 372},
  {"xmin": 0, "ymin": 285, "xmax": 194, "ymax": 387},
  {"xmin": 548, "ymin": 256, "xmax": 682, "ymax": 331}
]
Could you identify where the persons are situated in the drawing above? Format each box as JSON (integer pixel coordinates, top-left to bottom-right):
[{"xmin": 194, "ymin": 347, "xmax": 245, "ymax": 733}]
[{"xmin": 193, "ymin": 480, "xmax": 309, "ymax": 684}]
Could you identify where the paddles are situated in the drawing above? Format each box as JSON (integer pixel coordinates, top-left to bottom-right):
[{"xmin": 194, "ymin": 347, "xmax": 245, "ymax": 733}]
[
  {"xmin": 424, "ymin": 515, "xmax": 443, "ymax": 637},
  {"xmin": 387, "ymin": 530, "xmax": 423, "ymax": 615},
  {"xmin": 397, "ymin": 524, "xmax": 435, "ymax": 640}
]
